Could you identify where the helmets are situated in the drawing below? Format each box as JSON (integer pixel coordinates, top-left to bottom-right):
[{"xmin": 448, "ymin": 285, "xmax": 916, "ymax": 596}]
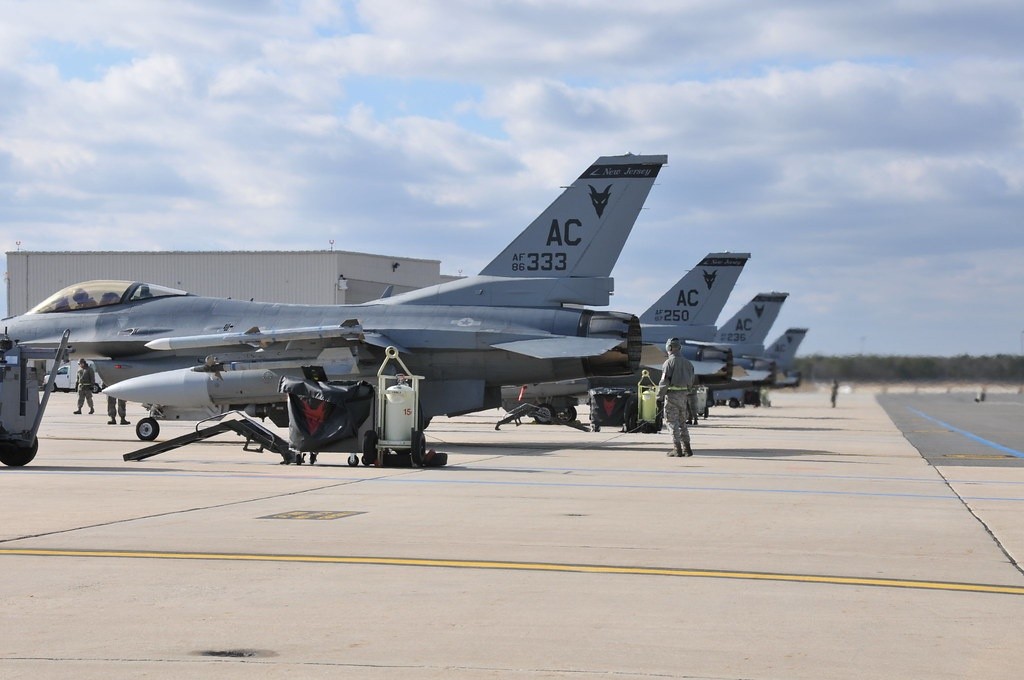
[
  {"xmin": 73, "ymin": 288, "xmax": 88, "ymax": 302},
  {"xmin": 77, "ymin": 358, "xmax": 86, "ymax": 364},
  {"xmin": 99, "ymin": 292, "xmax": 118, "ymax": 305}
]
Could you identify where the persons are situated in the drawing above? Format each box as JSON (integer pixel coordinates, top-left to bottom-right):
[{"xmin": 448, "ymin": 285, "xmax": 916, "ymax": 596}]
[
  {"xmin": 656, "ymin": 337, "xmax": 694, "ymax": 458},
  {"xmin": 101, "ymin": 381, "xmax": 131, "ymax": 425},
  {"xmin": 72, "ymin": 358, "xmax": 95, "ymax": 415},
  {"xmin": 831, "ymin": 376, "xmax": 839, "ymax": 409},
  {"xmin": 72, "ymin": 287, "xmax": 99, "ymax": 309}
]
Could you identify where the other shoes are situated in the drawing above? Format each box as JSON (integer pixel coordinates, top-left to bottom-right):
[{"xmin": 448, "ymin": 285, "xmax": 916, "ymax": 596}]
[
  {"xmin": 89, "ymin": 409, "xmax": 94, "ymax": 414},
  {"xmin": 108, "ymin": 420, "xmax": 116, "ymax": 424},
  {"xmin": 73, "ymin": 411, "xmax": 81, "ymax": 414},
  {"xmin": 120, "ymin": 420, "xmax": 130, "ymax": 424},
  {"xmin": 686, "ymin": 420, "xmax": 692, "ymax": 424},
  {"xmin": 680, "ymin": 448, "xmax": 692, "ymax": 456},
  {"xmin": 693, "ymin": 420, "xmax": 698, "ymax": 425},
  {"xmin": 667, "ymin": 450, "xmax": 681, "ymax": 457}
]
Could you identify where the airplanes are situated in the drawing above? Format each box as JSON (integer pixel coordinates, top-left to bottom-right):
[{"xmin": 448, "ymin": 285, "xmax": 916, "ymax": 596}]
[{"xmin": 1, "ymin": 151, "xmax": 812, "ymax": 473}]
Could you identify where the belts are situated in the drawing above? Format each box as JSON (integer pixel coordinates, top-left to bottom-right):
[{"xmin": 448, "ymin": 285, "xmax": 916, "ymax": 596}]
[{"xmin": 79, "ymin": 383, "xmax": 91, "ymax": 385}]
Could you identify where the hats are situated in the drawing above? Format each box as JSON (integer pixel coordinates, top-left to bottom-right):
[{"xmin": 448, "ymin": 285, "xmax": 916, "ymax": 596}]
[{"xmin": 666, "ymin": 338, "xmax": 680, "ymax": 348}]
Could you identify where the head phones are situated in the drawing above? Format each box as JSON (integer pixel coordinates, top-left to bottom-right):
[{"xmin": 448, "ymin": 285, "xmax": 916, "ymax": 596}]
[{"xmin": 666, "ymin": 337, "xmax": 681, "ymax": 351}]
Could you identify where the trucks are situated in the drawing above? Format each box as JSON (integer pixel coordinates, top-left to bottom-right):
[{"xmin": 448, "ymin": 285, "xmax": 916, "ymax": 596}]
[{"xmin": 41, "ymin": 361, "xmax": 103, "ymax": 394}]
